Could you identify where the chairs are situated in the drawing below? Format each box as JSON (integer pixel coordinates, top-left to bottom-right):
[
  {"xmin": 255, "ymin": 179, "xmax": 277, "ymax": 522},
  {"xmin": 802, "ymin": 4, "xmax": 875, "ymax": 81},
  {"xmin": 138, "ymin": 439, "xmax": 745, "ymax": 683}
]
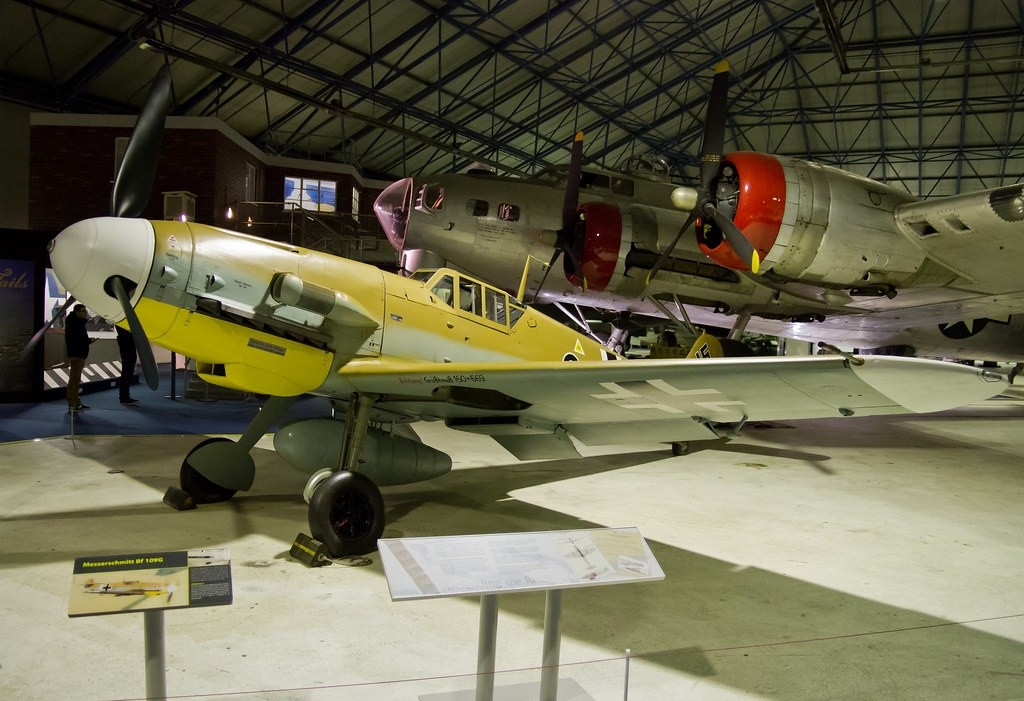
[{"xmin": 469, "ymin": 287, "xmax": 494, "ymax": 321}]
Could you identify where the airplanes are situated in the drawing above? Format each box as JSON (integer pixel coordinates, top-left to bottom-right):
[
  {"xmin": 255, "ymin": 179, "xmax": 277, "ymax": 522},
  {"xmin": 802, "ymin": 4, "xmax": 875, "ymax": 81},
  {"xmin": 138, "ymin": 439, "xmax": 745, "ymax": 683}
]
[
  {"xmin": 532, "ymin": 132, "xmax": 872, "ymax": 343},
  {"xmin": 17, "ymin": 65, "xmax": 1009, "ymax": 558},
  {"xmin": 373, "ymin": 155, "xmax": 879, "ymax": 357},
  {"xmin": 644, "ymin": 57, "xmax": 1022, "ymax": 337}
]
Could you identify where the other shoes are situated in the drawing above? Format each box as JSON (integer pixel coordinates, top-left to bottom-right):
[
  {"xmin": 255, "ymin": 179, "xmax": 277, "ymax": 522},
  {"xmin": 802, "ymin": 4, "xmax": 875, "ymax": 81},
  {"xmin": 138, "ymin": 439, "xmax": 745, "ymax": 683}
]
[
  {"xmin": 120, "ymin": 397, "xmax": 140, "ymax": 405},
  {"xmin": 68, "ymin": 403, "xmax": 91, "ymax": 411}
]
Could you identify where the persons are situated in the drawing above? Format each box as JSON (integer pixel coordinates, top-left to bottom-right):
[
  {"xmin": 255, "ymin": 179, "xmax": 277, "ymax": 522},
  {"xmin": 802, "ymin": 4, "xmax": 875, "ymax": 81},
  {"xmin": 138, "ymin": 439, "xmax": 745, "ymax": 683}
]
[
  {"xmin": 64, "ymin": 304, "xmax": 96, "ymax": 412},
  {"xmin": 115, "ymin": 325, "xmax": 140, "ymax": 404}
]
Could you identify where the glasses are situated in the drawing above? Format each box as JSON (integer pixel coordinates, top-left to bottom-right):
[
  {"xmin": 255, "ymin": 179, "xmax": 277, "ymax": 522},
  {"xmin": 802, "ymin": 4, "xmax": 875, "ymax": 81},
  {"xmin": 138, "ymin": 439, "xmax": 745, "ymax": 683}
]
[{"xmin": 77, "ymin": 310, "xmax": 87, "ymax": 313}]
